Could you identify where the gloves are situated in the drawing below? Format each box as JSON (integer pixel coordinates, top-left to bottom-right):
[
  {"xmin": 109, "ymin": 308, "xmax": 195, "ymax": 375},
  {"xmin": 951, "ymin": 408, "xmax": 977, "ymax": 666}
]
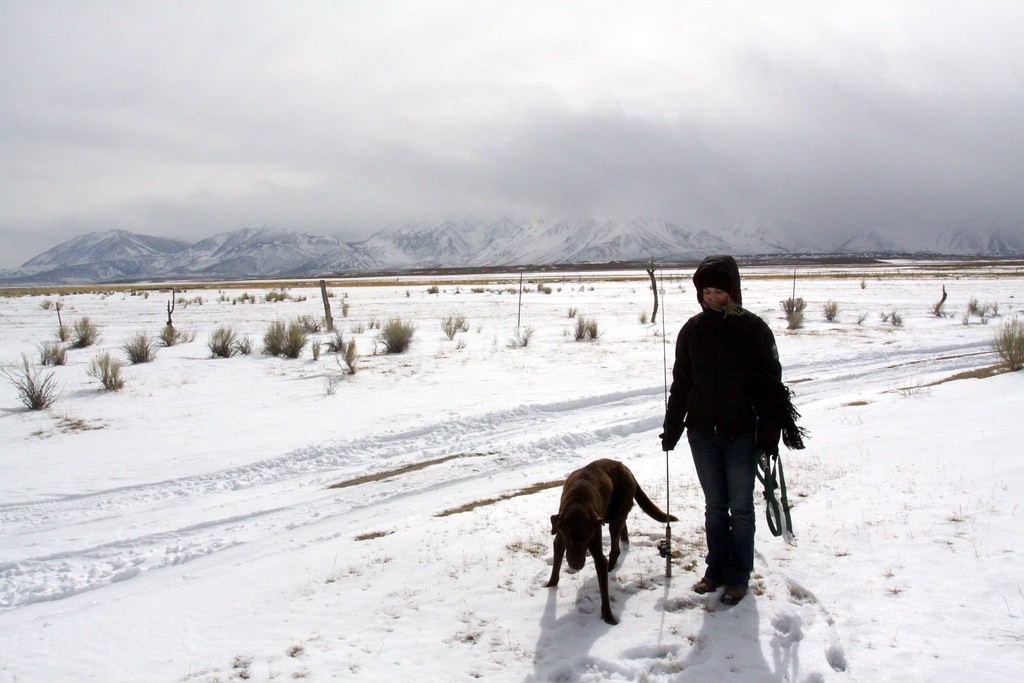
[
  {"xmin": 759, "ymin": 419, "xmax": 781, "ymax": 460},
  {"xmin": 659, "ymin": 412, "xmax": 684, "ymax": 451}
]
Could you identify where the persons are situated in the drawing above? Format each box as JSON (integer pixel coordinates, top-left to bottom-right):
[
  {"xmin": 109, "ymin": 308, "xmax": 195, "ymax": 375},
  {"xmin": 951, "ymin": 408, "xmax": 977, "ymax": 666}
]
[{"xmin": 663, "ymin": 255, "xmax": 796, "ymax": 605}]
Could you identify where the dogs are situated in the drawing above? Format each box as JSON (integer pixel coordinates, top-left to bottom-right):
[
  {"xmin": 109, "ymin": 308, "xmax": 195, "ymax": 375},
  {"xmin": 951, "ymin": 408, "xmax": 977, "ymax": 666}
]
[{"xmin": 545, "ymin": 458, "xmax": 679, "ymax": 625}]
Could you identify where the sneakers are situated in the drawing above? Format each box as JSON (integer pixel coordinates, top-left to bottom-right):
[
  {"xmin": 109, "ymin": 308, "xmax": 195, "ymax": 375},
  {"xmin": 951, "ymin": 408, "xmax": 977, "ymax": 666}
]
[
  {"xmin": 720, "ymin": 584, "xmax": 747, "ymax": 606},
  {"xmin": 692, "ymin": 578, "xmax": 717, "ymax": 595}
]
